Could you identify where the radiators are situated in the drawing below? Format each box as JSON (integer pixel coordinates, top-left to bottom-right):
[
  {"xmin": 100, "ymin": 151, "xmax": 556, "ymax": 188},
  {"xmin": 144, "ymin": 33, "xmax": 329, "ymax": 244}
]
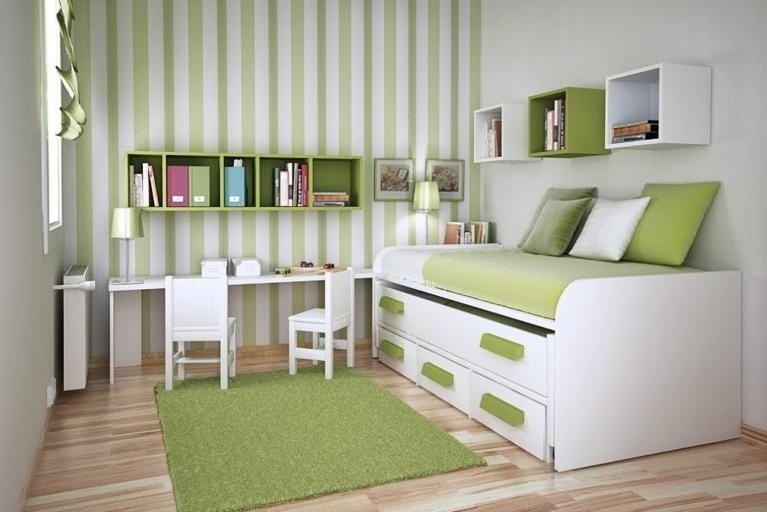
[{"xmin": 61, "ymin": 261, "xmax": 94, "ymax": 389}]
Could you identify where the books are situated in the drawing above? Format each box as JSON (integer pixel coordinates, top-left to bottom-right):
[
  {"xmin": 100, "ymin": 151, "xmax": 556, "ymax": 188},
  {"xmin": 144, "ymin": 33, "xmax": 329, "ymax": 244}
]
[
  {"xmin": 312, "ymin": 192, "xmax": 350, "ymax": 206},
  {"xmin": 168, "ymin": 165, "xmax": 210, "ymax": 208},
  {"xmin": 444, "ymin": 221, "xmax": 489, "ymax": 246},
  {"xmin": 482, "ymin": 118, "xmax": 501, "ymax": 158},
  {"xmin": 128, "ymin": 161, "xmax": 160, "ymax": 207},
  {"xmin": 225, "ymin": 159, "xmax": 246, "ymax": 208},
  {"xmin": 544, "ymin": 100, "xmax": 565, "ymax": 150},
  {"xmin": 612, "ymin": 119, "xmax": 658, "ymax": 140},
  {"xmin": 275, "ymin": 163, "xmax": 308, "ymax": 208}
]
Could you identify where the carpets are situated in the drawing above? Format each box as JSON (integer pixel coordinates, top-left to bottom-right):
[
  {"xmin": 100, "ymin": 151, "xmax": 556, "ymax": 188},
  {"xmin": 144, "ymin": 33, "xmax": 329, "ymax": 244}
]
[{"xmin": 153, "ymin": 363, "xmax": 488, "ymax": 511}]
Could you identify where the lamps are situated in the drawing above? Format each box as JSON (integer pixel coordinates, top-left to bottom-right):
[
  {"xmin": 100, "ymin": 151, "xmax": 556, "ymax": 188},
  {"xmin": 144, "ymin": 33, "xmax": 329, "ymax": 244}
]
[{"xmin": 413, "ymin": 181, "xmax": 441, "ymax": 246}]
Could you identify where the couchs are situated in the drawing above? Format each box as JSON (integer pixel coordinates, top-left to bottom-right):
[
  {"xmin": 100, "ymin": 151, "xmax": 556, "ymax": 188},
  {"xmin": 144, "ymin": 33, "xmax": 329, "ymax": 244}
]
[{"xmin": 372, "ymin": 242, "xmax": 741, "ymax": 472}]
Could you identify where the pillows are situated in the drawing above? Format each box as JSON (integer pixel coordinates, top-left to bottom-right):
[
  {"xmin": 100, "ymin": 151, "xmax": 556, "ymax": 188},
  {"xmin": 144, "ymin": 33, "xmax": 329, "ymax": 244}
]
[
  {"xmin": 621, "ymin": 180, "xmax": 723, "ymax": 267},
  {"xmin": 517, "ymin": 186, "xmax": 596, "ymax": 249},
  {"xmin": 522, "ymin": 197, "xmax": 589, "ymax": 257},
  {"xmin": 569, "ymin": 196, "xmax": 648, "ymax": 262}
]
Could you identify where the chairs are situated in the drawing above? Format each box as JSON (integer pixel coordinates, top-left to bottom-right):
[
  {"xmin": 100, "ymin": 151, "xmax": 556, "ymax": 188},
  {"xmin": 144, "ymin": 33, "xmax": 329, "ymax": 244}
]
[
  {"xmin": 164, "ymin": 275, "xmax": 237, "ymax": 391},
  {"xmin": 288, "ymin": 267, "xmax": 355, "ymax": 379}
]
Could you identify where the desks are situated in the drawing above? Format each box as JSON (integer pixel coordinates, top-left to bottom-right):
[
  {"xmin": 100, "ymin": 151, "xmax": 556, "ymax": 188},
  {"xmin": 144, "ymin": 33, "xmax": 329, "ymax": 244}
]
[{"xmin": 109, "ymin": 268, "xmax": 379, "ymax": 385}]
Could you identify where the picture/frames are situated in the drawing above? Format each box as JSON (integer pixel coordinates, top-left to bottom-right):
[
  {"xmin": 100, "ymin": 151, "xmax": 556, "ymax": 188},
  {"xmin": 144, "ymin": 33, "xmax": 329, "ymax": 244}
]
[
  {"xmin": 374, "ymin": 158, "xmax": 414, "ymax": 202},
  {"xmin": 425, "ymin": 159, "xmax": 465, "ymax": 201}
]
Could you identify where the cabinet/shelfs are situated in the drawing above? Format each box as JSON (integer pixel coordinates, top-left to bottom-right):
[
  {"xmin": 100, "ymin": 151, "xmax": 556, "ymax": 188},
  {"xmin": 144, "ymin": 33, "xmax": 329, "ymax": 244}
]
[
  {"xmin": 123, "ymin": 150, "xmax": 366, "ymax": 213},
  {"xmin": 470, "ymin": 60, "xmax": 714, "ymax": 163}
]
